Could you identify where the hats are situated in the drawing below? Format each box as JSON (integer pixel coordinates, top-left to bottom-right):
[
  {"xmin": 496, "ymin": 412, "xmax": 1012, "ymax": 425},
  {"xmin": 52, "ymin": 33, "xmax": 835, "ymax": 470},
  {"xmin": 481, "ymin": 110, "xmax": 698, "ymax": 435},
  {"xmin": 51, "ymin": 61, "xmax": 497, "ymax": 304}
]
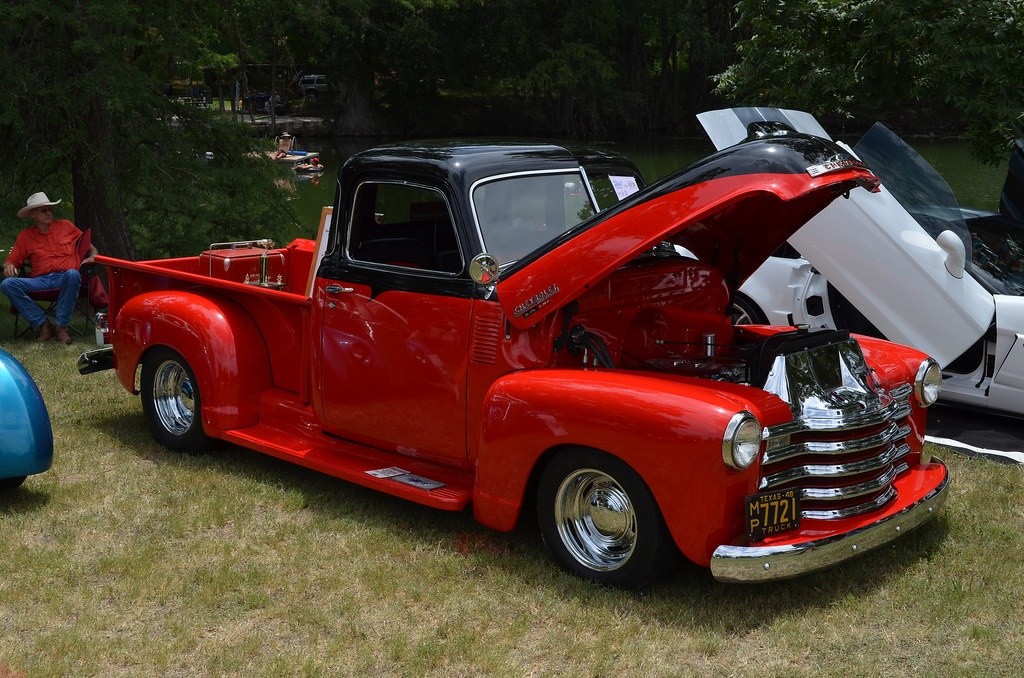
[
  {"xmin": 17, "ymin": 192, "xmax": 61, "ymax": 219},
  {"xmin": 280, "ymin": 132, "xmax": 292, "ymax": 137},
  {"xmin": 310, "ymin": 157, "xmax": 319, "ymax": 163}
]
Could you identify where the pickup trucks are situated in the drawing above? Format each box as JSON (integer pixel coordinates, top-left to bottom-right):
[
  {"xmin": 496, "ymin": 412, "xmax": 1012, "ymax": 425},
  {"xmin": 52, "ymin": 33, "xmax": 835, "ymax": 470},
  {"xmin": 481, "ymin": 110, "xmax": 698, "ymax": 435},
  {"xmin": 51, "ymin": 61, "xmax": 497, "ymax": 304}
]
[{"xmin": 76, "ymin": 120, "xmax": 949, "ymax": 587}]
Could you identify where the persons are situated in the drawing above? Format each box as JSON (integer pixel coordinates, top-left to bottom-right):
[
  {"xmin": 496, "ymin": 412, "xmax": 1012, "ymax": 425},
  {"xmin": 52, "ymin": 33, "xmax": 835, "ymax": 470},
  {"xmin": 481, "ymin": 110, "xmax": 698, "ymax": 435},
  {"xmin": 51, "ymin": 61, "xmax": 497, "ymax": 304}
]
[
  {"xmin": 195, "ymin": 93, "xmax": 207, "ymax": 109},
  {"xmin": 270, "ymin": 132, "xmax": 295, "ymax": 160},
  {"xmin": 265, "ymin": 90, "xmax": 288, "ymax": 115},
  {"xmin": 291, "ymin": 157, "xmax": 325, "ymax": 170},
  {"xmin": 1, "ymin": 192, "xmax": 100, "ymax": 345}
]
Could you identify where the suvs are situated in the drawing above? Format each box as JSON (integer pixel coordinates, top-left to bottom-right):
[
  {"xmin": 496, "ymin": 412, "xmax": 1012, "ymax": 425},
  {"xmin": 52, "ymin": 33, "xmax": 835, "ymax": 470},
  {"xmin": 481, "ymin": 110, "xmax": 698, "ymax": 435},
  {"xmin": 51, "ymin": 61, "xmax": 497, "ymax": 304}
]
[{"xmin": 298, "ymin": 74, "xmax": 339, "ymax": 98}]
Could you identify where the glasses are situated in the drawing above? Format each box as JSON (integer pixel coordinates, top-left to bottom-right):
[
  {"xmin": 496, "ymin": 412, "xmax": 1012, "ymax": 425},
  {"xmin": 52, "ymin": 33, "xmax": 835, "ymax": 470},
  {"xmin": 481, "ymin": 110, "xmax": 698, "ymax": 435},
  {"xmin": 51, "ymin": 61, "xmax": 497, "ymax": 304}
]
[{"xmin": 35, "ymin": 207, "xmax": 53, "ymax": 214}]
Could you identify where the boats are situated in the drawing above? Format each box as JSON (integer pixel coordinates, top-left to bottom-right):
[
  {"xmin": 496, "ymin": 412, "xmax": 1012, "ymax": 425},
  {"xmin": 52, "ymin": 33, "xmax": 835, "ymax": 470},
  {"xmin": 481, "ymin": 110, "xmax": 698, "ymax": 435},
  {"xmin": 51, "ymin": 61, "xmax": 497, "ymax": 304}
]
[{"xmin": 238, "ymin": 149, "xmax": 324, "ymax": 173}]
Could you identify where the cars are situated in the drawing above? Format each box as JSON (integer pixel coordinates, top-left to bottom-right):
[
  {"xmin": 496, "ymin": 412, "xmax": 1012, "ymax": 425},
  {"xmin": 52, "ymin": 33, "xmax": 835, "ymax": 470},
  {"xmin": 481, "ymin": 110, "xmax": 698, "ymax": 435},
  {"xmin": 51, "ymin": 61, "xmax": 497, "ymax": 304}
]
[{"xmin": 674, "ymin": 107, "xmax": 1024, "ymax": 427}]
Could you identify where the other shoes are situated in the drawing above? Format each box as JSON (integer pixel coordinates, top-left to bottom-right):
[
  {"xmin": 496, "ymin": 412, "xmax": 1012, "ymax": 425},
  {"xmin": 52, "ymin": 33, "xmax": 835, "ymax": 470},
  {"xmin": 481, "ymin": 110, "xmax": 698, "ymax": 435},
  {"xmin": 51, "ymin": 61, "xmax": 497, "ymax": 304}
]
[
  {"xmin": 37, "ymin": 321, "xmax": 55, "ymax": 342},
  {"xmin": 54, "ymin": 324, "xmax": 71, "ymax": 344}
]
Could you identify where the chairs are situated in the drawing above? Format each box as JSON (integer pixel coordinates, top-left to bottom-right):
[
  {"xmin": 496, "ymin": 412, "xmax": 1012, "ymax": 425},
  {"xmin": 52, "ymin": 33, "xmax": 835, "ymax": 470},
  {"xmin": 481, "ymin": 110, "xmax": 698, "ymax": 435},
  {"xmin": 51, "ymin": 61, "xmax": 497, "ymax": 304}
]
[{"xmin": 0, "ymin": 252, "xmax": 109, "ymax": 341}]
